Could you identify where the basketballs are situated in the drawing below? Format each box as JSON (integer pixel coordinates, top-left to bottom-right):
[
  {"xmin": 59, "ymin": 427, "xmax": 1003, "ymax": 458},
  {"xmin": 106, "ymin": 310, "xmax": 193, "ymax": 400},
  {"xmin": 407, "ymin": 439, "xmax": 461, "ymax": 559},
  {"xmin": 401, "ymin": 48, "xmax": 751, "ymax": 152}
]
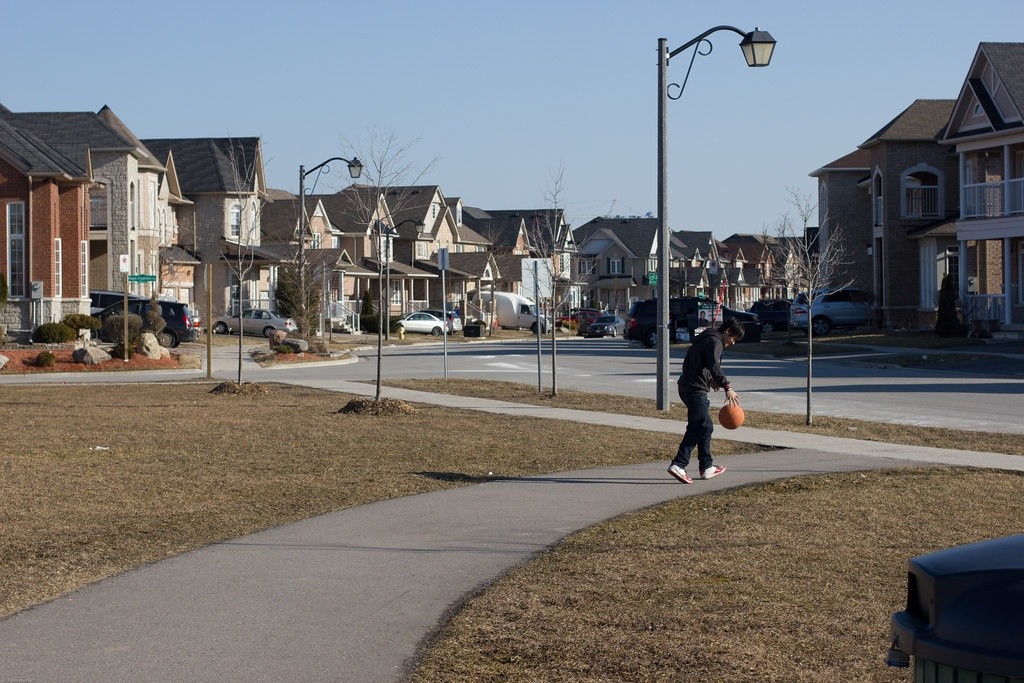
[{"xmin": 719, "ymin": 403, "xmax": 745, "ymax": 429}]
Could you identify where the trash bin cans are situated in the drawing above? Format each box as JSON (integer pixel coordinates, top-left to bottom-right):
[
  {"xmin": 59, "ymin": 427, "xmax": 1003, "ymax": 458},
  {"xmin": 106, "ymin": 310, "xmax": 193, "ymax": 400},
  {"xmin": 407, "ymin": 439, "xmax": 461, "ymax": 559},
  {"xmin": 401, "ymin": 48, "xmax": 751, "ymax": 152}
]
[{"xmin": 884, "ymin": 533, "xmax": 1024, "ymax": 683}]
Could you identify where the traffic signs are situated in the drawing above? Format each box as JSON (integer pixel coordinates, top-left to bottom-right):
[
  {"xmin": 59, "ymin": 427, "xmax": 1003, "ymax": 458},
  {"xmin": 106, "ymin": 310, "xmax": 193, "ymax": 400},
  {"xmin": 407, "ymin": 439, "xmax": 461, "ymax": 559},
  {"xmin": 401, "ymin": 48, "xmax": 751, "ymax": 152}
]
[{"xmin": 129, "ymin": 276, "xmax": 157, "ymax": 282}]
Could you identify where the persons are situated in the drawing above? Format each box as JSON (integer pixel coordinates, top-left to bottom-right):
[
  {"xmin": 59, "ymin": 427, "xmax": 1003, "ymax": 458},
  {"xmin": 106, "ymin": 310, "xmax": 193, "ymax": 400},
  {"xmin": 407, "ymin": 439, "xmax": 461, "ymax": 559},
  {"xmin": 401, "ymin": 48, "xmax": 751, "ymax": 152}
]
[
  {"xmin": 668, "ymin": 316, "xmax": 745, "ymax": 483},
  {"xmin": 677, "ymin": 334, "xmax": 683, "ymax": 341},
  {"xmin": 698, "ymin": 311, "xmax": 708, "ymax": 327}
]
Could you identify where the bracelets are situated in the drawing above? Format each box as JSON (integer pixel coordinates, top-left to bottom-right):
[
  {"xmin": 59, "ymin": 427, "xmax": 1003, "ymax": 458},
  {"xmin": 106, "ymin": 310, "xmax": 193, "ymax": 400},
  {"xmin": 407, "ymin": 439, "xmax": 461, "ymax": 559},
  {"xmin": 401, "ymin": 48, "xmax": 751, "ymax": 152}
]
[{"xmin": 725, "ymin": 387, "xmax": 732, "ymax": 392}]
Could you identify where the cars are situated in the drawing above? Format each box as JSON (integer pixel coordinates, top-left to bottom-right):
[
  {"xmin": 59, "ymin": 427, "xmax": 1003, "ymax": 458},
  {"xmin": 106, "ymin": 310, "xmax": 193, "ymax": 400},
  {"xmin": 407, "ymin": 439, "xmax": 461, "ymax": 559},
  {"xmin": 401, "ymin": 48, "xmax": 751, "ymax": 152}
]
[
  {"xmin": 389, "ymin": 312, "xmax": 449, "ymax": 336},
  {"xmin": 587, "ymin": 315, "xmax": 627, "ymax": 337},
  {"xmin": 212, "ymin": 308, "xmax": 296, "ymax": 338}
]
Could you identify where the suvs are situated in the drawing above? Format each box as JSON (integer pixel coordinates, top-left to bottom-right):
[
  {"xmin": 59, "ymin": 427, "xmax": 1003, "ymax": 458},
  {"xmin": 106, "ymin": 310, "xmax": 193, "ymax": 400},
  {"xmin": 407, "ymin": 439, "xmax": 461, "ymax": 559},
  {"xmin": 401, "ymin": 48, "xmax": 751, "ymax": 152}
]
[
  {"xmin": 418, "ymin": 310, "xmax": 462, "ymax": 335},
  {"xmin": 626, "ymin": 298, "xmax": 764, "ymax": 348},
  {"xmin": 790, "ymin": 288, "xmax": 884, "ymax": 336},
  {"xmin": 89, "ymin": 291, "xmax": 144, "ymax": 314},
  {"xmin": 89, "ymin": 300, "xmax": 202, "ymax": 349},
  {"xmin": 750, "ymin": 299, "xmax": 795, "ymax": 334},
  {"xmin": 555, "ymin": 308, "xmax": 602, "ymax": 333}
]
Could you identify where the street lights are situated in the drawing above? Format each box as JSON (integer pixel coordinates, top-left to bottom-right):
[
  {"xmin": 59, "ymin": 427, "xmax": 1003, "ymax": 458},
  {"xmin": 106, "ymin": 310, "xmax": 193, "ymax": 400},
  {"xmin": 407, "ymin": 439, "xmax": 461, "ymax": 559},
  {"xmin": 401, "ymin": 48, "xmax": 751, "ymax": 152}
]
[
  {"xmin": 656, "ymin": 25, "xmax": 775, "ymax": 412},
  {"xmin": 298, "ymin": 157, "xmax": 362, "ymax": 314},
  {"xmin": 385, "ymin": 218, "xmax": 424, "ymax": 341}
]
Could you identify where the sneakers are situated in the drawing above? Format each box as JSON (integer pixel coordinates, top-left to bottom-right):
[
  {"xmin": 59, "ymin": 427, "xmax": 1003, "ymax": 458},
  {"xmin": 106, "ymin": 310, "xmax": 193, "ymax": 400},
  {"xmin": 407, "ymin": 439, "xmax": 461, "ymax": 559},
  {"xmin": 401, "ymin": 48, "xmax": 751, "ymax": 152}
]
[
  {"xmin": 667, "ymin": 465, "xmax": 693, "ymax": 484},
  {"xmin": 701, "ymin": 465, "xmax": 726, "ymax": 480}
]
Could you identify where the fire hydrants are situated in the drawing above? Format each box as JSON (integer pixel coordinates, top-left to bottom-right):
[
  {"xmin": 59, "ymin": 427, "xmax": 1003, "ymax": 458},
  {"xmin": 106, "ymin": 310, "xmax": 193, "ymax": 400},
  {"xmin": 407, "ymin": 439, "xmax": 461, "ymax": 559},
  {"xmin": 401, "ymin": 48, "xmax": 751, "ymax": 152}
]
[{"xmin": 398, "ymin": 326, "xmax": 407, "ymax": 340}]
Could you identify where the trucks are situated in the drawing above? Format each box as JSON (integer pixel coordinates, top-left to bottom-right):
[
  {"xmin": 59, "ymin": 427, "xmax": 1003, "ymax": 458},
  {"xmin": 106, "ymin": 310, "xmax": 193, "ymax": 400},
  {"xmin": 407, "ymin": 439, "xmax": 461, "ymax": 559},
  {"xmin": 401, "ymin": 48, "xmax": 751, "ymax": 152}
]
[{"xmin": 473, "ymin": 291, "xmax": 552, "ymax": 333}]
